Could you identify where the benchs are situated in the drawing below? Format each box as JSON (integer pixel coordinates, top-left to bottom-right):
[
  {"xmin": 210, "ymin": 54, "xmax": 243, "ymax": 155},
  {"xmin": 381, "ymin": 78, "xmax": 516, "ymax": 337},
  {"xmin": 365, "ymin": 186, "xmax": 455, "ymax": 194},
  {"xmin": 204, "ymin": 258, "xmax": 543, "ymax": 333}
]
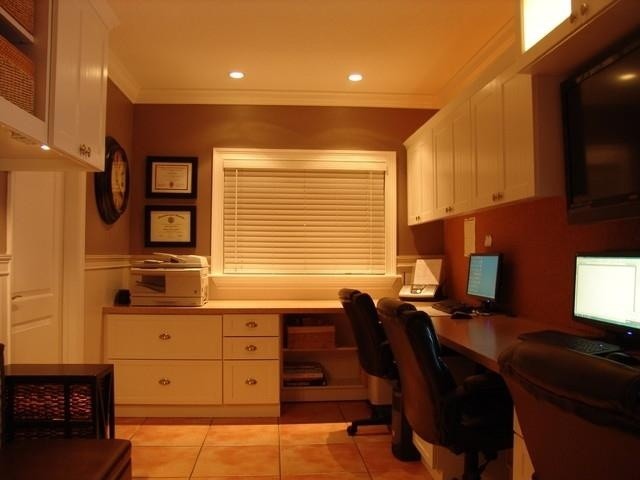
[{"xmin": 0, "ymin": 343, "xmax": 133, "ymax": 480}]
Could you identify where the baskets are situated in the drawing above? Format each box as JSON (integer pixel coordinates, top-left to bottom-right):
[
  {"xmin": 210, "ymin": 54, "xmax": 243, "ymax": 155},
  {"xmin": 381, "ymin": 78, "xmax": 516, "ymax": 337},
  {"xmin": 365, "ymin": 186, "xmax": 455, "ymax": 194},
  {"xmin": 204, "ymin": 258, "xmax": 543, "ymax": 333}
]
[
  {"xmin": 0, "ymin": 0, "xmax": 36, "ymax": 36},
  {"xmin": 0, "ymin": 36, "xmax": 35, "ymax": 114}
]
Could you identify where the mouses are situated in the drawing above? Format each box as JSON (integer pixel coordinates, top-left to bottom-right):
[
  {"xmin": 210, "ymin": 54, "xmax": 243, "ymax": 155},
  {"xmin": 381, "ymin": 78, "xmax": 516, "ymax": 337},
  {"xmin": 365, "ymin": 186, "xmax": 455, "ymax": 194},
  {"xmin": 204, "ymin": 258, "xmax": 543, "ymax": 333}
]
[
  {"xmin": 609, "ymin": 351, "xmax": 635, "ymax": 365},
  {"xmin": 450, "ymin": 311, "xmax": 472, "ymax": 319}
]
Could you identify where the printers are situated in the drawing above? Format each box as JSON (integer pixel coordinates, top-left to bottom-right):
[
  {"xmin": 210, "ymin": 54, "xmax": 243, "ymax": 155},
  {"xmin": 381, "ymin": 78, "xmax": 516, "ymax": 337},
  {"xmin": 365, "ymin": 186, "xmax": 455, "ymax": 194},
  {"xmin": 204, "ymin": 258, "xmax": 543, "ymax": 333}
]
[
  {"xmin": 399, "ymin": 258, "xmax": 444, "ymax": 301},
  {"xmin": 129, "ymin": 259, "xmax": 209, "ymax": 306}
]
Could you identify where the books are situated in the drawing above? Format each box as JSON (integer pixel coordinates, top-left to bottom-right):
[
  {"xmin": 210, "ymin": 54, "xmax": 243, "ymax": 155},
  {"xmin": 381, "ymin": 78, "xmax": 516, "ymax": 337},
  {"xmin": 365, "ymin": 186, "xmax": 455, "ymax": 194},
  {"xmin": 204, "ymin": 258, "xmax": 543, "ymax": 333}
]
[{"xmin": 283, "ymin": 362, "xmax": 327, "ymax": 387}]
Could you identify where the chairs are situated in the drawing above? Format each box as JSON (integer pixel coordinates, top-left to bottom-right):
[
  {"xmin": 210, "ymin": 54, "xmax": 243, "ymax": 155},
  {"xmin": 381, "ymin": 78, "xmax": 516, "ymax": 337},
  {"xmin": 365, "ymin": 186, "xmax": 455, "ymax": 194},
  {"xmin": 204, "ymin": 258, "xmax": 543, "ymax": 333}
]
[
  {"xmin": 377, "ymin": 298, "xmax": 510, "ymax": 479},
  {"xmin": 337, "ymin": 289, "xmax": 399, "ymax": 437},
  {"xmin": 498, "ymin": 341, "xmax": 639, "ymax": 480}
]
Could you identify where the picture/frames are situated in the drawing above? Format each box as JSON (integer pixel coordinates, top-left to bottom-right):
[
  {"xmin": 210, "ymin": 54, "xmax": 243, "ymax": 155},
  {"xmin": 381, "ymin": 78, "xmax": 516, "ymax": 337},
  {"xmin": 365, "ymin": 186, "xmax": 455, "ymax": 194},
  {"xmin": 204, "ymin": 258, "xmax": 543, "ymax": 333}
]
[
  {"xmin": 146, "ymin": 156, "xmax": 198, "ymax": 197},
  {"xmin": 143, "ymin": 206, "xmax": 197, "ymax": 247}
]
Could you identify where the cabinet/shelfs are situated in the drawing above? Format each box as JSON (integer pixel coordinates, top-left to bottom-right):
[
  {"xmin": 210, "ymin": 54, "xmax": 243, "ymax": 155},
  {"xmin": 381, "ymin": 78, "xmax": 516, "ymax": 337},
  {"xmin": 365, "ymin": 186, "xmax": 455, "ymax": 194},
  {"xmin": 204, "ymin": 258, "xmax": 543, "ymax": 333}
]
[
  {"xmin": 403, "ymin": 116, "xmax": 435, "ymax": 226},
  {"xmin": 102, "ymin": 308, "xmax": 222, "ymax": 418},
  {"xmin": 0, "ymin": 0, "xmax": 117, "ymax": 175},
  {"xmin": 435, "ymin": 90, "xmax": 477, "ymax": 219},
  {"xmin": 514, "ymin": 0, "xmax": 640, "ymax": 75},
  {"xmin": 411, "ymin": 300, "xmax": 640, "ymax": 479},
  {"xmin": 0, "ymin": 0, "xmax": 49, "ymax": 159},
  {"xmin": 476, "ymin": 65, "xmax": 564, "ymax": 211},
  {"xmin": 1, "ymin": 364, "xmax": 115, "ymax": 440},
  {"xmin": 222, "ymin": 308, "xmax": 282, "ymax": 417}
]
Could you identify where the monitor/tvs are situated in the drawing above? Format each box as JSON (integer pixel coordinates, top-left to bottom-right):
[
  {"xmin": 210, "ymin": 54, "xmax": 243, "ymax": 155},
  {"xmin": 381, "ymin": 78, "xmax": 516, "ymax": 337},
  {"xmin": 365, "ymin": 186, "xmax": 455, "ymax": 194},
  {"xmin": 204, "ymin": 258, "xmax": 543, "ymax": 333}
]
[
  {"xmin": 571, "ymin": 252, "xmax": 640, "ymax": 347},
  {"xmin": 559, "ymin": 36, "xmax": 640, "ymax": 224},
  {"xmin": 466, "ymin": 253, "xmax": 505, "ymax": 315}
]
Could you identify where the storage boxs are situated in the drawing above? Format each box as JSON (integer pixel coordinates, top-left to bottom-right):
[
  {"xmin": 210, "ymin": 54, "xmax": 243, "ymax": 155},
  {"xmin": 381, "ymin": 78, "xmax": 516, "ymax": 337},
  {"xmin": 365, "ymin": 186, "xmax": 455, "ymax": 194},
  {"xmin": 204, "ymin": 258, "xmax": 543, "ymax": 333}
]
[{"xmin": 287, "ymin": 325, "xmax": 336, "ymax": 349}]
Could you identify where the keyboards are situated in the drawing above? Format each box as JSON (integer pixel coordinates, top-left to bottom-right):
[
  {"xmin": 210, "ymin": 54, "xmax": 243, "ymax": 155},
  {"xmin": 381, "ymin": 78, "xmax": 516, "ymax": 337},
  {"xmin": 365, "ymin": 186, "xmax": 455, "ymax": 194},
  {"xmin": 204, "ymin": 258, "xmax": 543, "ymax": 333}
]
[
  {"xmin": 432, "ymin": 303, "xmax": 474, "ymax": 314},
  {"xmin": 518, "ymin": 330, "xmax": 622, "ymax": 355}
]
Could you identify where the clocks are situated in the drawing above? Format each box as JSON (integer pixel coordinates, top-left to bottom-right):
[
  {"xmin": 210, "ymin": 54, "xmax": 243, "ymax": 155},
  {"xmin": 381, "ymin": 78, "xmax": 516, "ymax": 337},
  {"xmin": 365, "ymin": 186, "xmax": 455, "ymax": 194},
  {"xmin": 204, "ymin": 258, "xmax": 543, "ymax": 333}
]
[{"xmin": 94, "ymin": 135, "xmax": 130, "ymax": 225}]
